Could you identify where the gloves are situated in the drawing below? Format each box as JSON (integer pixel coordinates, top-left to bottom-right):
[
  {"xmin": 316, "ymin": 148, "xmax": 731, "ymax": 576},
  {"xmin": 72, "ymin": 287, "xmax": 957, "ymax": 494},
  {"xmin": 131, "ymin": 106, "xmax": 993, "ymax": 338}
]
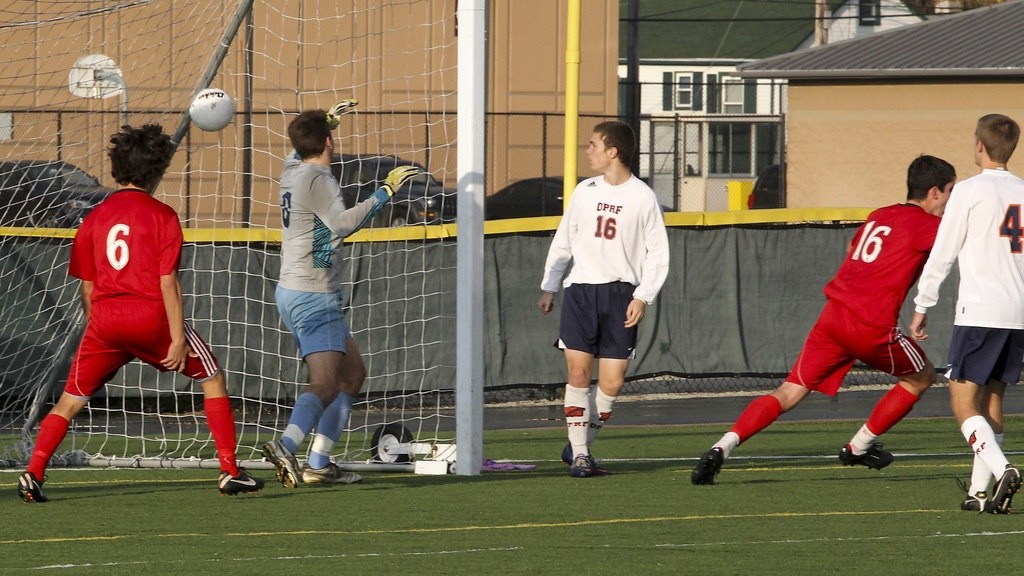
[
  {"xmin": 379, "ymin": 165, "xmax": 421, "ymax": 197},
  {"xmin": 325, "ymin": 98, "xmax": 359, "ymax": 129}
]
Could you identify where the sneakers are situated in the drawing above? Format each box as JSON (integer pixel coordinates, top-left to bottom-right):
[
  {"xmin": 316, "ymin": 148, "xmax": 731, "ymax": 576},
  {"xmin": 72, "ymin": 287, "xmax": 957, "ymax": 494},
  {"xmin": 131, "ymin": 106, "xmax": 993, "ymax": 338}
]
[
  {"xmin": 690, "ymin": 447, "xmax": 725, "ymax": 485},
  {"xmin": 216, "ymin": 466, "xmax": 264, "ymax": 496},
  {"xmin": 260, "ymin": 439, "xmax": 302, "ymax": 489},
  {"xmin": 984, "ymin": 463, "xmax": 1024, "ymax": 515},
  {"xmin": 838, "ymin": 442, "xmax": 895, "ymax": 471},
  {"xmin": 301, "ymin": 461, "xmax": 363, "ymax": 485},
  {"xmin": 955, "ymin": 475, "xmax": 989, "ymax": 514},
  {"xmin": 17, "ymin": 471, "xmax": 50, "ymax": 503}
]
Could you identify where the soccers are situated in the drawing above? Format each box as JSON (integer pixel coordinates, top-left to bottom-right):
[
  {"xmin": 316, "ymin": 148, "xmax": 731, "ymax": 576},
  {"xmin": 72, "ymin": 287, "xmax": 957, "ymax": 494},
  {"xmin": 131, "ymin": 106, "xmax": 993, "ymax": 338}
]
[{"xmin": 188, "ymin": 87, "xmax": 235, "ymax": 132}]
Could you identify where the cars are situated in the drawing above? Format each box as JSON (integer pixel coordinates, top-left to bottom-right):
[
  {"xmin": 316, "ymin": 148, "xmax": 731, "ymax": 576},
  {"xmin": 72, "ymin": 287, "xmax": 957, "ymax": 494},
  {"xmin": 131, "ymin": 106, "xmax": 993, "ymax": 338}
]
[
  {"xmin": 746, "ymin": 161, "xmax": 786, "ymax": 209},
  {"xmin": 486, "ymin": 174, "xmax": 676, "ymax": 225},
  {"xmin": 0, "ymin": 159, "xmax": 115, "ymax": 227}
]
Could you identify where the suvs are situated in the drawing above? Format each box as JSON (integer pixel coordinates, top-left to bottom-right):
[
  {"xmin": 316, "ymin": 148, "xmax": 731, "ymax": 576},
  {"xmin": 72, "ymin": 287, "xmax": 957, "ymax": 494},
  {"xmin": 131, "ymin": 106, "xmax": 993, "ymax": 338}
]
[{"xmin": 329, "ymin": 152, "xmax": 457, "ymax": 230}]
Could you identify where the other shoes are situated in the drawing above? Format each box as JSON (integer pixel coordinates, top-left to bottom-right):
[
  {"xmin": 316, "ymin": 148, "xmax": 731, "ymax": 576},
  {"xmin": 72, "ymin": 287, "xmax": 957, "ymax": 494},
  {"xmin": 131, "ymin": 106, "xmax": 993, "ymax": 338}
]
[
  {"xmin": 570, "ymin": 453, "xmax": 594, "ymax": 478},
  {"xmin": 561, "ymin": 442, "xmax": 573, "ymax": 465}
]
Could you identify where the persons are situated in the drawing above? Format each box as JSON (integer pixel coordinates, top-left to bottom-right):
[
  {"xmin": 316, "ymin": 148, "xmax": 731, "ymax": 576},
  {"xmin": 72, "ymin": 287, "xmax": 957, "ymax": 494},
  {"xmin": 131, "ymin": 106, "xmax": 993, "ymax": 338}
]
[
  {"xmin": 259, "ymin": 100, "xmax": 422, "ymax": 490},
  {"xmin": 690, "ymin": 151, "xmax": 956, "ymax": 486},
  {"xmin": 12, "ymin": 122, "xmax": 268, "ymax": 504},
  {"xmin": 910, "ymin": 112, "xmax": 1024, "ymax": 521},
  {"xmin": 535, "ymin": 120, "xmax": 671, "ymax": 479}
]
